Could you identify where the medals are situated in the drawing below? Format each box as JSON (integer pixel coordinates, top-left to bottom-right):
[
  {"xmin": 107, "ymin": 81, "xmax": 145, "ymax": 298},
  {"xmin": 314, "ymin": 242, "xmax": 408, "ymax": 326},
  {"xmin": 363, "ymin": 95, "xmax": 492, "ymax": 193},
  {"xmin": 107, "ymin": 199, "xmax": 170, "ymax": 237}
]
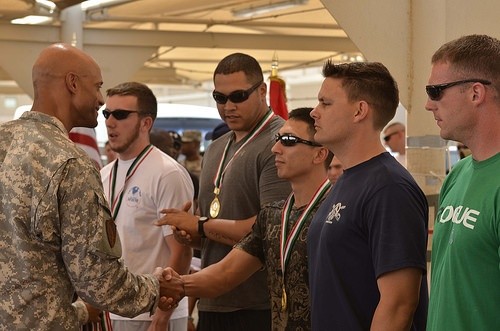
[
  {"xmin": 208, "ymin": 187, "xmax": 221, "ymax": 218},
  {"xmin": 281, "ymin": 284, "xmax": 287, "ymax": 311}
]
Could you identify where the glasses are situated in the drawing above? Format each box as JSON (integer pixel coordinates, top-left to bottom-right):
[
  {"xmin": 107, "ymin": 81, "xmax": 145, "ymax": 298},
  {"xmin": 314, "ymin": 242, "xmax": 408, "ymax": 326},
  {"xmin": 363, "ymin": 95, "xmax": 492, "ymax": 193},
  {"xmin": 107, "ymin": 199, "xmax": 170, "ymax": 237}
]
[
  {"xmin": 426, "ymin": 79, "xmax": 490, "ymax": 101},
  {"xmin": 213, "ymin": 81, "xmax": 262, "ymax": 105},
  {"xmin": 102, "ymin": 109, "xmax": 138, "ymax": 120},
  {"xmin": 168, "ymin": 132, "xmax": 179, "ymax": 139},
  {"xmin": 275, "ymin": 132, "xmax": 324, "ymax": 146},
  {"xmin": 384, "ymin": 132, "xmax": 399, "ymax": 141}
]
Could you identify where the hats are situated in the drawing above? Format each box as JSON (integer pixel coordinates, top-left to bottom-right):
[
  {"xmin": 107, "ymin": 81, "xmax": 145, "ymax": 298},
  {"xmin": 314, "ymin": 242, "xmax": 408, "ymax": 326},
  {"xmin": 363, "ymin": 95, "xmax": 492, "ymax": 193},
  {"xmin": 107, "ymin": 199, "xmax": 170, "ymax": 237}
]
[
  {"xmin": 168, "ymin": 131, "xmax": 182, "ymax": 146},
  {"xmin": 180, "ymin": 131, "xmax": 201, "ymax": 142},
  {"xmin": 205, "ymin": 123, "xmax": 229, "ymax": 141}
]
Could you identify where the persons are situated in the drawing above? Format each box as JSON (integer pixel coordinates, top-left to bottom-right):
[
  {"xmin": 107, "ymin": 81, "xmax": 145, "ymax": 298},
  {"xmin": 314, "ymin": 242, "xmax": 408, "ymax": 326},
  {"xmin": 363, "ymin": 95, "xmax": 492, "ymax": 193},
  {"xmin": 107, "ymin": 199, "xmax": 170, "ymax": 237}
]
[
  {"xmin": 103, "ymin": 119, "xmax": 234, "ymax": 164},
  {"xmin": 384, "ymin": 121, "xmax": 406, "ymax": 170},
  {"xmin": 77, "ymin": 81, "xmax": 195, "ymax": 331},
  {"xmin": 152, "ymin": 106, "xmax": 331, "ymax": 330},
  {"xmin": 174, "ymin": 128, "xmax": 205, "ymax": 217},
  {"xmin": 424, "ymin": 33, "xmax": 500, "ymax": 331},
  {"xmin": 0, "ymin": 43, "xmax": 181, "ymax": 330},
  {"xmin": 327, "ymin": 157, "xmax": 344, "ymax": 186},
  {"xmin": 309, "ymin": 59, "xmax": 431, "ymax": 331},
  {"xmin": 154, "ymin": 50, "xmax": 294, "ymax": 331}
]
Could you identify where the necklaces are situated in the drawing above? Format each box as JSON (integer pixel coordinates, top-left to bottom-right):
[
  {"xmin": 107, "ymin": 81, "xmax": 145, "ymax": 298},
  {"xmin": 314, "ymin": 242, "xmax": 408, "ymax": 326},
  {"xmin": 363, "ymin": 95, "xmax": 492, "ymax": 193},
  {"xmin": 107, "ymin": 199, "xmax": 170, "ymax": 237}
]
[{"xmin": 109, "ymin": 144, "xmax": 155, "ymax": 221}]
